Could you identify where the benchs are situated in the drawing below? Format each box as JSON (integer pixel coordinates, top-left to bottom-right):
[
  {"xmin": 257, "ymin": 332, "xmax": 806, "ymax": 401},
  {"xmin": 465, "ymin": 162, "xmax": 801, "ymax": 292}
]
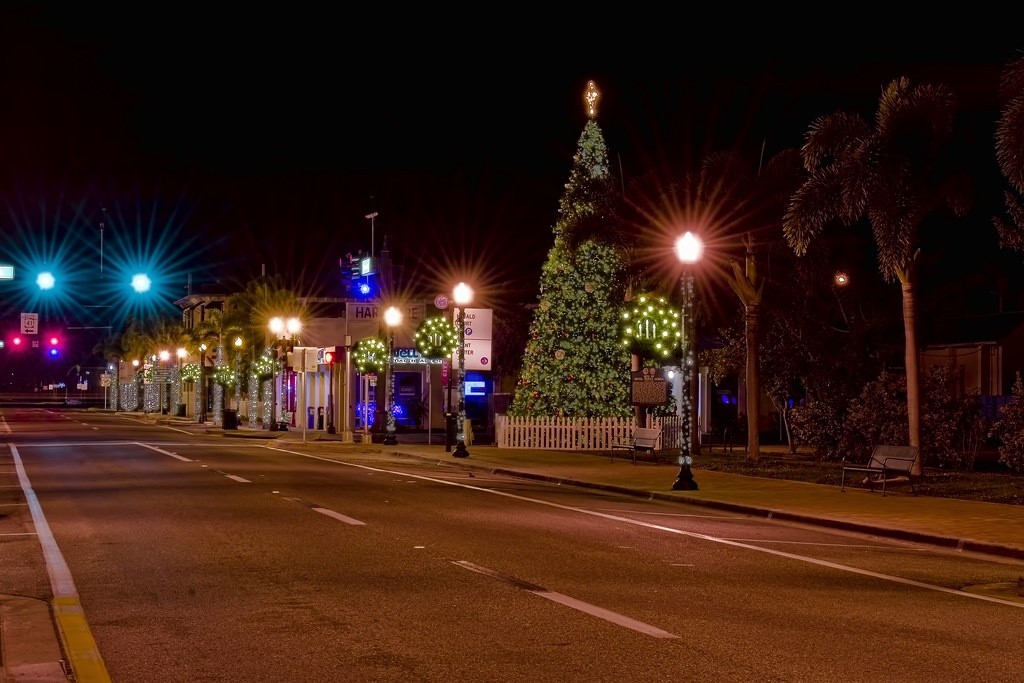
[
  {"xmin": 610, "ymin": 429, "xmax": 662, "ymax": 465},
  {"xmin": 842, "ymin": 445, "xmax": 921, "ymax": 496}
]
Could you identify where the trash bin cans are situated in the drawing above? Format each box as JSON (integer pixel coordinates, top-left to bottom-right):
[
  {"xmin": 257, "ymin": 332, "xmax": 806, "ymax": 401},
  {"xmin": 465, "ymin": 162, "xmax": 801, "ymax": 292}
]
[
  {"xmin": 177, "ymin": 404, "xmax": 186, "ymax": 417},
  {"xmin": 220, "ymin": 409, "xmax": 239, "ymax": 430},
  {"xmin": 374, "ymin": 409, "xmax": 388, "ymax": 432},
  {"xmin": 451, "ymin": 418, "xmax": 458, "ymax": 443},
  {"xmin": 318, "ymin": 407, "xmax": 324, "ymax": 430},
  {"xmin": 307, "ymin": 407, "xmax": 314, "ymax": 429}
]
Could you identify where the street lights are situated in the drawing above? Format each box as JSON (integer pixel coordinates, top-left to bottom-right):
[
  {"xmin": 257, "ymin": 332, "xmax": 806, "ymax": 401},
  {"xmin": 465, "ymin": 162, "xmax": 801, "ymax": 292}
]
[
  {"xmin": 451, "ymin": 282, "xmax": 474, "ymax": 457},
  {"xmin": 669, "ymin": 229, "xmax": 698, "ymax": 491},
  {"xmin": 382, "ymin": 306, "xmax": 403, "ymax": 446},
  {"xmin": 197, "ymin": 343, "xmax": 209, "ymax": 424},
  {"xmin": 266, "ymin": 316, "xmax": 302, "ymax": 432}
]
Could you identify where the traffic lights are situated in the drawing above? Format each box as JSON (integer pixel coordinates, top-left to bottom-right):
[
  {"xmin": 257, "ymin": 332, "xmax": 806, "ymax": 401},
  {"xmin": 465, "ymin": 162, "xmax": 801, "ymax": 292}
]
[
  {"xmin": 13, "ymin": 337, "xmax": 22, "ymax": 345},
  {"xmin": 50, "ymin": 338, "xmax": 59, "ymax": 356}
]
